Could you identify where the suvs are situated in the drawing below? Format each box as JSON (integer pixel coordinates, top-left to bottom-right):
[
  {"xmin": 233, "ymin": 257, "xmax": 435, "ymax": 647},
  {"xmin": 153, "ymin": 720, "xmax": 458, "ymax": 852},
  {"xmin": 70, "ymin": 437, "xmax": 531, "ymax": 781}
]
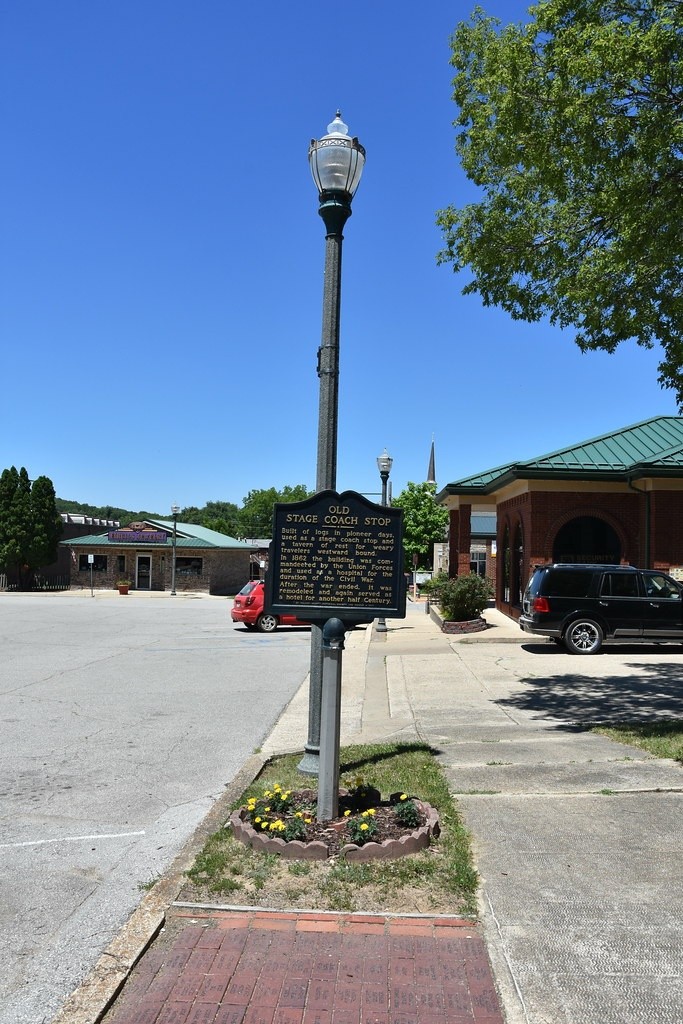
[
  {"xmin": 230, "ymin": 577, "xmax": 356, "ymax": 634},
  {"xmin": 518, "ymin": 561, "xmax": 683, "ymax": 655}
]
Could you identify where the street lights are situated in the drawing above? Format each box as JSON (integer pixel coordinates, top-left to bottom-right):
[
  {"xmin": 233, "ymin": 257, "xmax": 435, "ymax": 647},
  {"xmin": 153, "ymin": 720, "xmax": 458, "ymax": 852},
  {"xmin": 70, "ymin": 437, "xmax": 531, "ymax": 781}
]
[
  {"xmin": 291, "ymin": 108, "xmax": 367, "ymax": 775},
  {"xmin": 169, "ymin": 504, "xmax": 181, "ymax": 596},
  {"xmin": 374, "ymin": 446, "xmax": 395, "ymax": 633}
]
